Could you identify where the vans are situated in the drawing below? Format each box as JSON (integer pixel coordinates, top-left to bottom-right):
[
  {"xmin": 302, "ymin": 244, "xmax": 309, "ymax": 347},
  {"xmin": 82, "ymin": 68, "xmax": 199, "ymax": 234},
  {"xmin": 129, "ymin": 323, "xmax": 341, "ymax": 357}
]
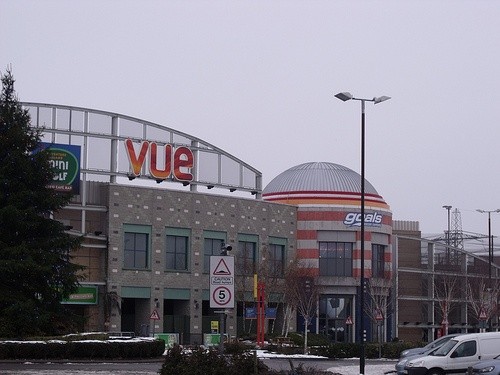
[{"xmin": 404, "ymin": 332, "xmax": 500, "ymax": 375}]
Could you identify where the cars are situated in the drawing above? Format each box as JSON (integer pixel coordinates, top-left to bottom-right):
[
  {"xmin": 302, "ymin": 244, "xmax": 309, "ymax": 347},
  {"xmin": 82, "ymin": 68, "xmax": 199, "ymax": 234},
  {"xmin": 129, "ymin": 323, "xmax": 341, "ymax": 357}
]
[
  {"xmin": 400, "ymin": 334, "xmax": 459, "ymax": 358},
  {"xmin": 394, "ymin": 341, "xmax": 445, "ymax": 375},
  {"xmin": 467, "ymin": 354, "xmax": 500, "ymax": 375}
]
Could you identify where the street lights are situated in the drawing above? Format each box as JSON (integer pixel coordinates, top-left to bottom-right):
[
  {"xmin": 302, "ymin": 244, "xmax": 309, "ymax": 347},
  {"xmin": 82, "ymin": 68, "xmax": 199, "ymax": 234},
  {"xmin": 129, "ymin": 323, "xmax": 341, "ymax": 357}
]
[
  {"xmin": 475, "ymin": 209, "xmax": 500, "ymax": 332},
  {"xmin": 334, "ymin": 91, "xmax": 392, "ymax": 375}
]
